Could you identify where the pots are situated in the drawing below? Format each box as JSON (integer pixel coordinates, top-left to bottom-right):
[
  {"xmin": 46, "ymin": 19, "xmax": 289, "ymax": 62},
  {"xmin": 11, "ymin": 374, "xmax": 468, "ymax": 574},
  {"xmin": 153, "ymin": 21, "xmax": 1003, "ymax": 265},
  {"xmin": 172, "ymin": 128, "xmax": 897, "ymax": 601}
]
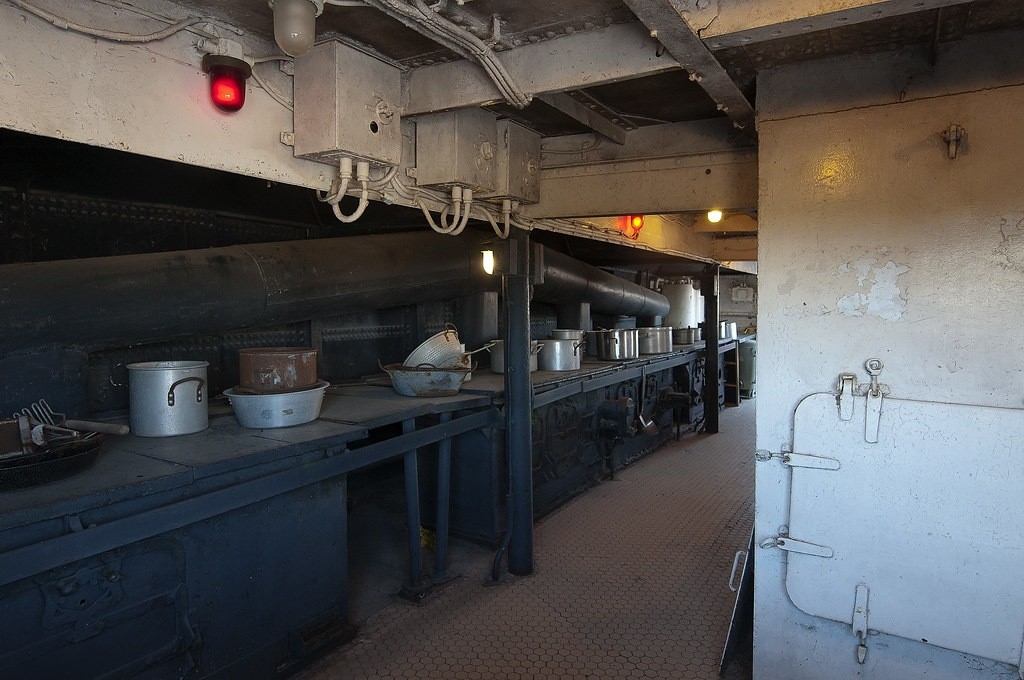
[
  {"xmin": 489, "ymin": 321, "xmax": 736, "ymax": 374},
  {"xmin": 0, "ymin": 433, "xmax": 105, "ymax": 488},
  {"xmin": 376, "ymin": 323, "xmax": 478, "ymax": 397},
  {"xmin": 126, "ymin": 361, "xmax": 210, "ymax": 437},
  {"xmin": 215, "ymin": 347, "xmax": 330, "ymax": 428}
]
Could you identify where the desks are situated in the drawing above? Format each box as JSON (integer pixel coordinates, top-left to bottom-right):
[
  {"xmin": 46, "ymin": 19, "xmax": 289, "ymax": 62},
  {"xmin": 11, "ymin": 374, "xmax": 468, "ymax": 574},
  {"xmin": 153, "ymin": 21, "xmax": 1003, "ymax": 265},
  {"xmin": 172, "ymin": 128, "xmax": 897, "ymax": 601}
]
[{"xmin": 0, "ymin": 330, "xmax": 764, "ymax": 680}]
